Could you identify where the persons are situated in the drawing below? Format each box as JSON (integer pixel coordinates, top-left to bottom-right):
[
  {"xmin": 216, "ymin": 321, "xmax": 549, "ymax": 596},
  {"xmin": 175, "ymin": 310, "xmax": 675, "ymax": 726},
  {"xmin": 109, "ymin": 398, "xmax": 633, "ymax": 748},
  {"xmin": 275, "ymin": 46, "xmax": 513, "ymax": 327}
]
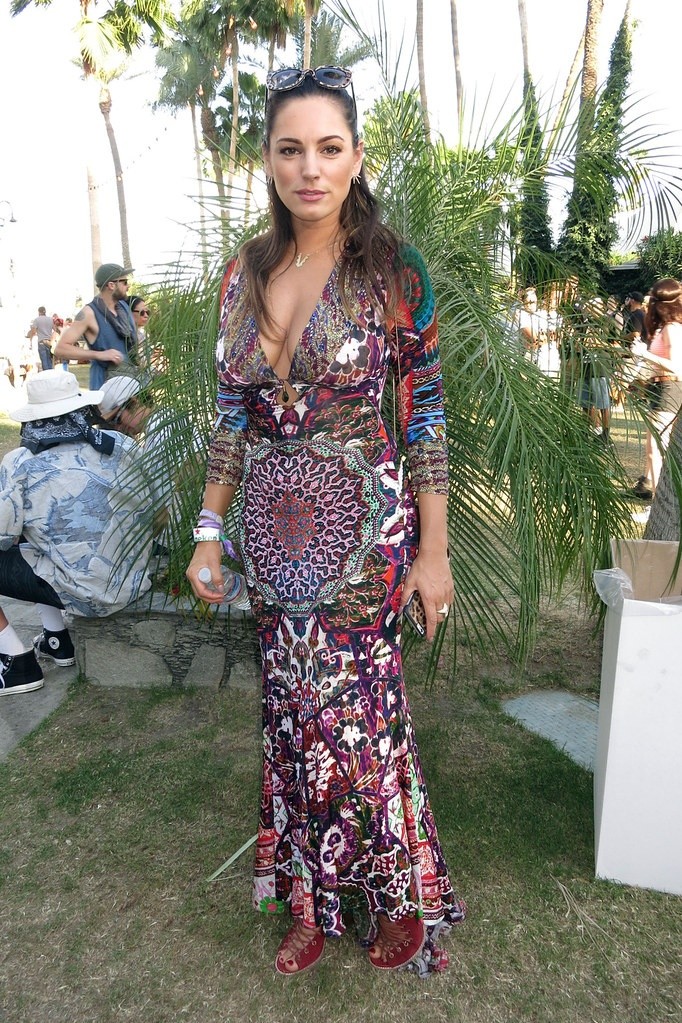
[
  {"xmin": 0, "ymin": 262, "xmax": 206, "ymax": 696},
  {"xmin": 509, "ymin": 279, "xmax": 682, "ymax": 501},
  {"xmin": 186, "ymin": 63, "xmax": 465, "ymax": 975}
]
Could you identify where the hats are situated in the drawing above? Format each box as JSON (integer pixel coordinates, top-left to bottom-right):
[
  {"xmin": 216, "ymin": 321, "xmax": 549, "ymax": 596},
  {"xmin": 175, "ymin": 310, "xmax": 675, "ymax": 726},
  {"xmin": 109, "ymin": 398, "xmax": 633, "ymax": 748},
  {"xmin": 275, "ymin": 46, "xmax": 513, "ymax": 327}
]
[
  {"xmin": 10, "ymin": 369, "xmax": 105, "ymax": 423},
  {"xmin": 95, "ymin": 263, "xmax": 135, "ymax": 291},
  {"xmin": 628, "ymin": 292, "xmax": 644, "ymax": 303},
  {"xmin": 96, "ymin": 376, "xmax": 143, "ymax": 416}
]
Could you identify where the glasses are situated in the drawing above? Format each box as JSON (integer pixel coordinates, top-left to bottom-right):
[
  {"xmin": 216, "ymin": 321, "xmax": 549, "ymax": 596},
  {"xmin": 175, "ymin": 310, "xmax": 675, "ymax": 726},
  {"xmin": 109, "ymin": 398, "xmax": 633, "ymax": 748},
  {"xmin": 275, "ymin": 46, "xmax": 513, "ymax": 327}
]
[
  {"xmin": 264, "ymin": 64, "xmax": 357, "ymax": 135},
  {"xmin": 106, "ymin": 279, "xmax": 128, "ymax": 286},
  {"xmin": 132, "ymin": 310, "xmax": 150, "ymax": 316},
  {"xmin": 105, "ymin": 398, "xmax": 132, "ymax": 427}
]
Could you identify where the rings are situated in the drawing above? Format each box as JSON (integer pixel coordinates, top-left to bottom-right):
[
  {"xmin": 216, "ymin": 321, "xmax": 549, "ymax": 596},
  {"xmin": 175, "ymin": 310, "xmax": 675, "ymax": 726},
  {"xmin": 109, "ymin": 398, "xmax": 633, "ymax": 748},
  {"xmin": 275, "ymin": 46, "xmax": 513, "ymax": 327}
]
[
  {"xmin": 437, "ymin": 603, "xmax": 450, "ymax": 617},
  {"xmin": 116, "ymin": 355, "xmax": 120, "ymax": 360}
]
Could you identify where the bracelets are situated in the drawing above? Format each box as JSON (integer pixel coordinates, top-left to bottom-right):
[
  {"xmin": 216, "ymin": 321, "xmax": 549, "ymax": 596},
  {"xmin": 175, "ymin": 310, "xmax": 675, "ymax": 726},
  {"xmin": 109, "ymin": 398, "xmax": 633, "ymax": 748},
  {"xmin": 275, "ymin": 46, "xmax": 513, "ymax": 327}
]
[{"xmin": 192, "ymin": 509, "xmax": 237, "ymax": 558}]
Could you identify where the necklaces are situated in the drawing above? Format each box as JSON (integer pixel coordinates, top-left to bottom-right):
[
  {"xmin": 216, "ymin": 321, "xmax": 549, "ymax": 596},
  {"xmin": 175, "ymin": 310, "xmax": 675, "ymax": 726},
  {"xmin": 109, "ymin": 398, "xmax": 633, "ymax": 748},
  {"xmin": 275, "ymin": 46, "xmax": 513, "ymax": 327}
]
[{"xmin": 290, "ymin": 240, "xmax": 339, "ymax": 267}]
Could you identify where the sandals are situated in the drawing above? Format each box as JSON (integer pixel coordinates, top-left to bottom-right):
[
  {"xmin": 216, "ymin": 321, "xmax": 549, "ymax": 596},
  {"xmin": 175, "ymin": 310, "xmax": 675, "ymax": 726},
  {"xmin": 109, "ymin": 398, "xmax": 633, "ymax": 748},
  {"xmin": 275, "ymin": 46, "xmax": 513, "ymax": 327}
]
[
  {"xmin": 369, "ymin": 912, "xmax": 426, "ymax": 970},
  {"xmin": 274, "ymin": 918, "xmax": 328, "ymax": 974}
]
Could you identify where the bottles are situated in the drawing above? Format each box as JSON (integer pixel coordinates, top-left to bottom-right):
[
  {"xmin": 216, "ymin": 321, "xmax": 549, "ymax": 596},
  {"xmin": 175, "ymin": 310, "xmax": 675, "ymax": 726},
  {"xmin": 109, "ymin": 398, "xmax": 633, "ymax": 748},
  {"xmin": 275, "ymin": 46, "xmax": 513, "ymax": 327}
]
[{"xmin": 198, "ymin": 564, "xmax": 252, "ymax": 610}]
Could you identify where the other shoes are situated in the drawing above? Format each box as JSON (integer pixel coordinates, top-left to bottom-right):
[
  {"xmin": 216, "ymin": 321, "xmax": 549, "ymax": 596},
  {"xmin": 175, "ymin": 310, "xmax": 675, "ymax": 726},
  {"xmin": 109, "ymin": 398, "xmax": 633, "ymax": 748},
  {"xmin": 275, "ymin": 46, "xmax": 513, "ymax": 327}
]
[
  {"xmin": 594, "ymin": 434, "xmax": 615, "ymax": 446},
  {"xmin": 624, "ymin": 475, "xmax": 653, "ymax": 500}
]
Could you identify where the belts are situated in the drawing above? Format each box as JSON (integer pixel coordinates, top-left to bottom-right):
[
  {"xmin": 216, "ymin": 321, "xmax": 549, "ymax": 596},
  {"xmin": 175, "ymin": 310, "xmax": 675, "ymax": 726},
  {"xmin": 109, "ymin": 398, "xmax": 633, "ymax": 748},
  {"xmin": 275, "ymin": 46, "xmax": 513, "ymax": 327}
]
[{"xmin": 648, "ymin": 375, "xmax": 677, "ymax": 383}]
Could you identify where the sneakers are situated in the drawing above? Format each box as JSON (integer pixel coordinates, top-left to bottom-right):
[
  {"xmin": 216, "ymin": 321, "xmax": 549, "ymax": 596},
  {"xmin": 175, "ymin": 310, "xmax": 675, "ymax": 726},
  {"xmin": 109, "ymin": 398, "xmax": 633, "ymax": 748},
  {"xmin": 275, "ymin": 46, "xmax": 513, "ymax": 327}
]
[
  {"xmin": 32, "ymin": 628, "xmax": 76, "ymax": 667},
  {"xmin": 0, "ymin": 647, "xmax": 45, "ymax": 697}
]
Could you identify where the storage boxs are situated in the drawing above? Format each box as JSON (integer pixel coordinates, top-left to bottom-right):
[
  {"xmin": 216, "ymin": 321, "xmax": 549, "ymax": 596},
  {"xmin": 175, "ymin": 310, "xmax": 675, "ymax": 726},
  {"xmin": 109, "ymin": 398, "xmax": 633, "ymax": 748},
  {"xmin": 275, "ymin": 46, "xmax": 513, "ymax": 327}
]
[{"xmin": 592, "ymin": 539, "xmax": 680, "ymax": 897}]
